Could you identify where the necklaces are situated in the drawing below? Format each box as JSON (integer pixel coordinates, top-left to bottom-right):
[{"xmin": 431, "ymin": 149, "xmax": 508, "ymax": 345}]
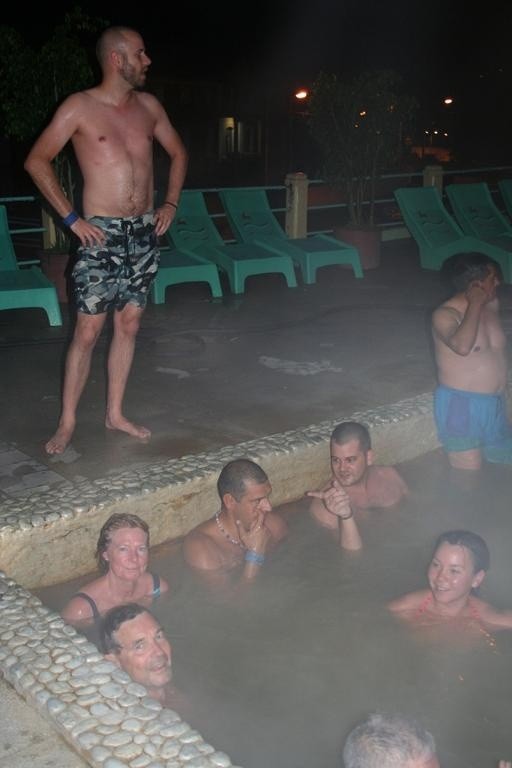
[{"xmin": 216, "ymin": 511, "xmax": 242, "ymax": 544}]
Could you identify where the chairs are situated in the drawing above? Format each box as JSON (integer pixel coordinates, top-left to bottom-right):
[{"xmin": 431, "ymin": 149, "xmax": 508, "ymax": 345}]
[
  {"xmin": 392, "ymin": 185, "xmax": 512, "ymax": 284},
  {"xmin": 0, "ymin": 205, "xmax": 67, "ymax": 329},
  {"xmin": 444, "ymin": 182, "xmax": 512, "ymax": 251},
  {"xmin": 497, "ymin": 178, "xmax": 512, "ymax": 217},
  {"xmin": 157, "ymin": 187, "xmax": 299, "ymax": 293},
  {"xmin": 219, "ymin": 189, "xmax": 365, "ymax": 284}
]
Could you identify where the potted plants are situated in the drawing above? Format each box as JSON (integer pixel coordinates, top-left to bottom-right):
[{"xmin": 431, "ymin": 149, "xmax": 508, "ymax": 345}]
[
  {"xmin": 290, "ymin": 68, "xmax": 419, "ymax": 268},
  {"xmin": 0, "ymin": 6, "xmax": 107, "ymax": 303}
]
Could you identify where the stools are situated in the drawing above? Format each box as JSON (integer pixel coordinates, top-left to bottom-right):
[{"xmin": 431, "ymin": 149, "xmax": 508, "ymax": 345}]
[{"xmin": 149, "ymin": 247, "xmax": 224, "ymax": 304}]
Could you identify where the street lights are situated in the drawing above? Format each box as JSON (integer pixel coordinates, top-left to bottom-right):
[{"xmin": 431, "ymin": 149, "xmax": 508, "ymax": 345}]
[{"xmin": 435, "ymin": 94, "xmax": 455, "ymax": 148}]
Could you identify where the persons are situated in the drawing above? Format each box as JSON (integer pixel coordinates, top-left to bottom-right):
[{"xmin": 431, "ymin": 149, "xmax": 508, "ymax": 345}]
[
  {"xmin": 430, "ymin": 252, "xmax": 512, "ymax": 471},
  {"xmin": 306, "ymin": 422, "xmax": 408, "ymax": 551},
  {"xmin": 342, "ymin": 714, "xmax": 512, "ymax": 768},
  {"xmin": 60, "ymin": 513, "xmax": 171, "ymax": 631},
  {"xmin": 182, "ymin": 460, "xmax": 291, "ymax": 585},
  {"xmin": 100, "ymin": 603, "xmax": 173, "ymax": 705},
  {"xmin": 383, "ymin": 531, "xmax": 512, "ymax": 635},
  {"xmin": 22, "ymin": 27, "xmax": 191, "ymax": 454}
]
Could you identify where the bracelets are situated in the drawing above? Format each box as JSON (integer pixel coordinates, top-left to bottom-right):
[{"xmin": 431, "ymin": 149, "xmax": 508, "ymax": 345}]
[
  {"xmin": 339, "ymin": 510, "xmax": 352, "ymax": 520},
  {"xmin": 163, "ymin": 202, "xmax": 177, "ymax": 209},
  {"xmin": 62, "ymin": 209, "xmax": 80, "ymax": 227},
  {"xmin": 244, "ymin": 549, "xmax": 267, "ymax": 568}
]
